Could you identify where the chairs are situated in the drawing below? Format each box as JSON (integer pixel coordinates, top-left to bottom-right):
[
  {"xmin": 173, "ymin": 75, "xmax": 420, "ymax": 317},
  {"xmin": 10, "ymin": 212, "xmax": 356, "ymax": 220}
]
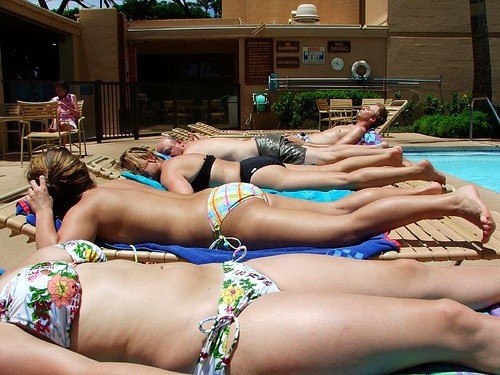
[
  {"xmin": 361, "ymin": 99, "xmax": 384, "ymax": 105},
  {"xmin": 17, "ymin": 101, "xmax": 66, "ymax": 168},
  {"xmin": 164, "ymin": 101, "xmax": 174, "ymax": 122},
  {"xmin": 0, "ymin": 122, "xmax": 480, "ymax": 259},
  {"xmin": 316, "ymin": 99, "xmax": 351, "ymax": 130},
  {"xmin": 176, "ymin": 100, "xmax": 193, "ymax": 123},
  {"xmin": 331, "ymin": 99, "xmax": 353, "ymax": 128},
  {"xmin": 67, "ymin": 100, "xmax": 87, "ymax": 156}
]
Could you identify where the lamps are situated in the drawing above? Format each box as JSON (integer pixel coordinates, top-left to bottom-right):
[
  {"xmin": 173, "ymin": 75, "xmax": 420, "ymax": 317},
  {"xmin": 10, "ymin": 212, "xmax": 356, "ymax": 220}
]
[{"xmin": 291, "ymin": 4, "xmax": 320, "ymax": 24}]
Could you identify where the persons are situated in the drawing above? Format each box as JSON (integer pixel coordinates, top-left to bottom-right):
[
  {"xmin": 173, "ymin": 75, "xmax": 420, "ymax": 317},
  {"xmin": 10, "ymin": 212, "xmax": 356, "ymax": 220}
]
[
  {"xmin": 120, "ymin": 145, "xmax": 447, "ymax": 195},
  {"xmin": 23, "ymin": 147, "xmax": 497, "ymax": 251},
  {"xmin": 0, "ymin": 240, "xmax": 500, "ymax": 375},
  {"xmin": 283, "ymin": 103, "xmax": 388, "ymax": 145},
  {"xmin": 43, "ymin": 80, "xmax": 81, "ymax": 148},
  {"xmin": 156, "ymin": 134, "xmax": 411, "ymax": 167}
]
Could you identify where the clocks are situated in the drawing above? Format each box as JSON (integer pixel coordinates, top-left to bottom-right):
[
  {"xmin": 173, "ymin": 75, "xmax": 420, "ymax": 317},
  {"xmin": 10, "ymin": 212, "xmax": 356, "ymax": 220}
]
[{"xmin": 331, "ymin": 57, "xmax": 344, "ymax": 71}]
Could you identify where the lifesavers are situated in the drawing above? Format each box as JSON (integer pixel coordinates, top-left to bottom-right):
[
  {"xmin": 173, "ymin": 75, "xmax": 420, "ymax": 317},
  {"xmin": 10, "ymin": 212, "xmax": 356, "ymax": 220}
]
[{"xmin": 350, "ymin": 59, "xmax": 372, "ymax": 79}]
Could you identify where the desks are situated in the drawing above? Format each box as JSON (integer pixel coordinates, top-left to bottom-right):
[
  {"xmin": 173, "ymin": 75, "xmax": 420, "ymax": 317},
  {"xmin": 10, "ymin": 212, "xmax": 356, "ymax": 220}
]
[{"xmin": 191, "ymin": 105, "xmax": 208, "ymax": 123}]
